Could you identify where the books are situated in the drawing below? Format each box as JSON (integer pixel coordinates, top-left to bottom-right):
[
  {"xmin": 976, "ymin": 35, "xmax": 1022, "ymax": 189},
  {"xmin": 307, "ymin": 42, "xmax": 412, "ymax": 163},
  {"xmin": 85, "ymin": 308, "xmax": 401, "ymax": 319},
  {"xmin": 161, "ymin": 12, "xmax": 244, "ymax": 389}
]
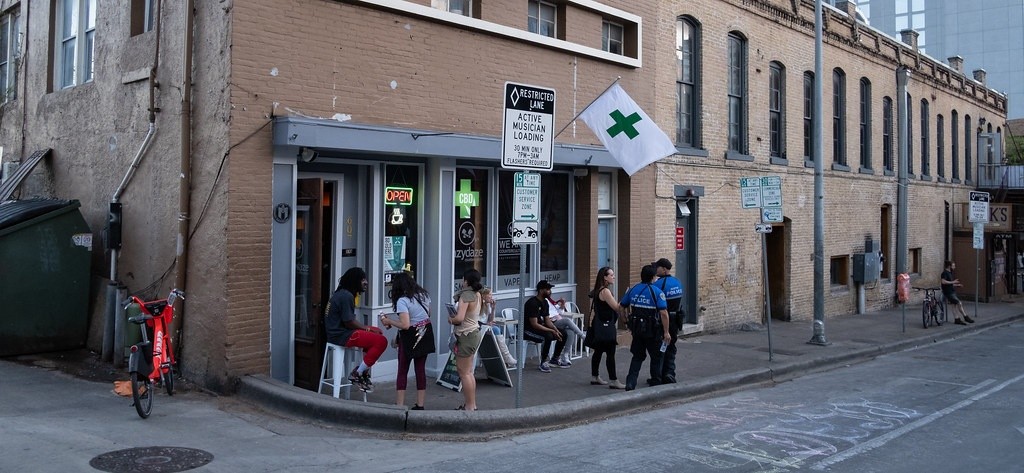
[{"xmin": 446, "ymin": 303, "xmax": 462, "ymax": 326}]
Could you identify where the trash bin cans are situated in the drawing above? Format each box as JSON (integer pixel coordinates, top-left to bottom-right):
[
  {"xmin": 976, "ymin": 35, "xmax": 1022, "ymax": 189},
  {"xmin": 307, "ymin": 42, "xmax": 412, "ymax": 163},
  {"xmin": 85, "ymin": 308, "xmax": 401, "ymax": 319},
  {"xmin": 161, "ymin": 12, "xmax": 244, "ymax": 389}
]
[{"xmin": 0, "ymin": 199, "xmax": 93, "ymax": 358}]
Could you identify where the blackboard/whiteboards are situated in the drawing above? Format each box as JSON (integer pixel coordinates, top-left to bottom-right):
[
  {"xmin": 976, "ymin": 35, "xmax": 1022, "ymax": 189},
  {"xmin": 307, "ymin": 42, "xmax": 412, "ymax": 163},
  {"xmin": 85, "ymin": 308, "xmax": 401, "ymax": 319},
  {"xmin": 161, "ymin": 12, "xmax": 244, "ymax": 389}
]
[{"xmin": 436, "ymin": 324, "xmax": 514, "ymax": 390}]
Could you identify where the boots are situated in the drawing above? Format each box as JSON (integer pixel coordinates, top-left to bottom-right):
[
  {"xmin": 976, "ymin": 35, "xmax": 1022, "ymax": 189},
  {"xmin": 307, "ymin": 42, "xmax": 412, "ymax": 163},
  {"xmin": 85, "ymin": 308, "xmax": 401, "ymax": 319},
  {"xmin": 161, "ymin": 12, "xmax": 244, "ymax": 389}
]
[{"xmin": 496, "ymin": 335, "xmax": 518, "ymax": 364}]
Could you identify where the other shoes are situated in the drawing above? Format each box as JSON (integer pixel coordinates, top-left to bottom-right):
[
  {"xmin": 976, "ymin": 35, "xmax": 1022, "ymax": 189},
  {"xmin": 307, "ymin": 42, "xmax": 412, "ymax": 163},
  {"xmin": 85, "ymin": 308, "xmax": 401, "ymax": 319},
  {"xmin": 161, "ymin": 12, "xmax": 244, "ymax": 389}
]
[
  {"xmin": 647, "ymin": 378, "xmax": 652, "ymax": 384},
  {"xmin": 964, "ymin": 315, "xmax": 975, "ymax": 323},
  {"xmin": 412, "ymin": 403, "xmax": 424, "ymax": 410},
  {"xmin": 609, "ymin": 378, "xmax": 626, "ymax": 389},
  {"xmin": 455, "ymin": 404, "xmax": 478, "ymax": 411},
  {"xmin": 664, "ymin": 374, "xmax": 677, "ymax": 384},
  {"xmin": 591, "ymin": 376, "xmax": 609, "ymax": 385},
  {"xmin": 954, "ymin": 317, "xmax": 967, "ymax": 325}
]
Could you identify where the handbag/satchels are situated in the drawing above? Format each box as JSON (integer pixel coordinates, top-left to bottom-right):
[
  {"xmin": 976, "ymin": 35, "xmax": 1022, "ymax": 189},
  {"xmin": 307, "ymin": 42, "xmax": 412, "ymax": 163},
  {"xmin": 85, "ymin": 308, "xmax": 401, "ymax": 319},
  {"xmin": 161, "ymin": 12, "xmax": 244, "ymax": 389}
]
[
  {"xmin": 583, "ymin": 327, "xmax": 595, "ymax": 349},
  {"xmin": 592, "ymin": 316, "xmax": 619, "ymax": 347},
  {"xmin": 399, "ymin": 319, "xmax": 436, "ymax": 359}
]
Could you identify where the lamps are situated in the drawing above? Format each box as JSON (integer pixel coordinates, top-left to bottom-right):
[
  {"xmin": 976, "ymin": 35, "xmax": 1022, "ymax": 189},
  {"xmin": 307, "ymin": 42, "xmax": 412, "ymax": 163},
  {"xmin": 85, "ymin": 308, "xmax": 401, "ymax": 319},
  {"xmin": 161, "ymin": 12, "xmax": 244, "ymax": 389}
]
[{"xmin": 297, "ymin": 146, "xmax": 318, "ymax": 163}]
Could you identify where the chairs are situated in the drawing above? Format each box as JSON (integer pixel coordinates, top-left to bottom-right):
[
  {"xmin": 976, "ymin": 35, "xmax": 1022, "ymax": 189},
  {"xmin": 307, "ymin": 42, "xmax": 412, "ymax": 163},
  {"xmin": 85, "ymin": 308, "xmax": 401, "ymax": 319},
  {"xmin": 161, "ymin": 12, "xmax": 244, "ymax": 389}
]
[
  {"xmin": 502, "ymin": 308, "xmax": 542, "ymax": 369},
  {"xmin": 563, "ymin": 302, "xmax": 589, "ymax": 357}
]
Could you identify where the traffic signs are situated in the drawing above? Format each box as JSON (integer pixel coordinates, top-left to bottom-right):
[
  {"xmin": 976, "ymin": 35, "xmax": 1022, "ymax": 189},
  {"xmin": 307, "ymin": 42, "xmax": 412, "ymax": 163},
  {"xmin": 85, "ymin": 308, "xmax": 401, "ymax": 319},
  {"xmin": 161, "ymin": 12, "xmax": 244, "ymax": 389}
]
[
  {"xmin": 514, "ymin": 173, "xmax": 540, "ymax": 222},
  {"xmin": 740, "ymin": 177, "xmax": 762, "ymax": 209},
  {"xmin": 762, "ymin": 207, "xmax": 784, "ymax": 223},
  {"xmin": 760, "ymin": 175, "xmax": 783, "ymax": 208}
]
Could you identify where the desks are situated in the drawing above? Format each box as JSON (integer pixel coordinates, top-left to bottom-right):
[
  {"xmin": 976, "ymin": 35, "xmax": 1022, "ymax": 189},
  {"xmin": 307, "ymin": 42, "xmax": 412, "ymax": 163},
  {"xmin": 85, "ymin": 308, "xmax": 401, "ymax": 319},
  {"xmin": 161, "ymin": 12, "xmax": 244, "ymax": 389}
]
[
  {"xmin": 564, "ymin": 313, "xmax": 584, "ymax": 360},
  {"xmin": 494, "ymin": 317, "xmax": 518, "ymax": 358}
]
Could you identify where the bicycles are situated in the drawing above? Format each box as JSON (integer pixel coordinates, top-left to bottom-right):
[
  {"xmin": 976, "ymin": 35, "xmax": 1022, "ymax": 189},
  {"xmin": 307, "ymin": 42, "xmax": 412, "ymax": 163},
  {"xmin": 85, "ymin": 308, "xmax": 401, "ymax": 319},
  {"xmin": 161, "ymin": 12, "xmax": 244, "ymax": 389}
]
[
  {"xmin": 120, "ymin": 288, "xmax": 186, "ymax": 419},
  {"xmin": 912, "ymin": 286, "xmax": 945, "ymax": 330}
]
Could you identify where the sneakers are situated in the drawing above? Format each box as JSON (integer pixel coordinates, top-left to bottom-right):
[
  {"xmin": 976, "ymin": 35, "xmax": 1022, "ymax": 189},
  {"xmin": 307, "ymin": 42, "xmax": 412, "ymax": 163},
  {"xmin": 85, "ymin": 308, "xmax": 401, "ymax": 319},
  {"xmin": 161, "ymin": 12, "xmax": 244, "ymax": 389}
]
[
  {"xmin": 348, "ymin": 368, "xmax": 374, "ymax": 393},
  {"xmin": 539, "ymin": 360, "xmax": 552, "ymax": 373},
  {"xmin": 549, "ymin": 358, "xmax": 571, "ymax": 368}
]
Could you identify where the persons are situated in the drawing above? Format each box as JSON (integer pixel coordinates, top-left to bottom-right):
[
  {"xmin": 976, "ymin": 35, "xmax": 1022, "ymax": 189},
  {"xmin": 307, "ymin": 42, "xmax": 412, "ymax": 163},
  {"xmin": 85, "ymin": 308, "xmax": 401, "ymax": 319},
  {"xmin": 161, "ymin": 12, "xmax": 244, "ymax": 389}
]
[
  {"xmin": 941, "ymin": 261, "xmax": 975, "ymax": 325},
  {"xmin": 620, "ymin": 265, "xmax": 671, "ymax": 391},
  {"xmin": 545, "ymin": 296, "xmax": 586, "ymax": 365},
  {"xmin": 324, "ymin": 267, "xmax": 388, "ymax": 393},
  {"xmin": 647, "ymin": 258, "xmax": 683, "ymax": 384},
  {"xmin": 448, "ymin": 269, "xmax": 483, "ymax": 411},
  {"xmin": 382, "ymin": 272, "xmax": 432, "ymax": 410},
  {"xmin": 524, "ymin": 280, "xmax": 570, "ymax": 372},
  {"xmin": 479, "ymin": 285, "xmax": 517, "ymax": 365},
  {"xmin": 590, "ymin": 267, "xmax": 626, "ymax": 389}
]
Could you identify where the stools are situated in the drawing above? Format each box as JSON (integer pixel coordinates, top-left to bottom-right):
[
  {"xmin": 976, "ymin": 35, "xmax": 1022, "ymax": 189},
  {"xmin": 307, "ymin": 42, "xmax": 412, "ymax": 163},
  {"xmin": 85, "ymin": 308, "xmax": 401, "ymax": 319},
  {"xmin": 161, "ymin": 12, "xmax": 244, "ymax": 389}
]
[{"xmin": 317, "ymin": 342, "xmax": 368, "ymax": 403}]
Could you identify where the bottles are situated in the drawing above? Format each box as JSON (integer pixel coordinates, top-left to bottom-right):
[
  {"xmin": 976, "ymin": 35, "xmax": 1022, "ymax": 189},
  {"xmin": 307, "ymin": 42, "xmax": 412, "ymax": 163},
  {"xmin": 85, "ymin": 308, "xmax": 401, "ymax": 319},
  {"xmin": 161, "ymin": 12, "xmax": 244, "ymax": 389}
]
[
  {"xmin": 379, "ymin": 310, "xmax": 392, "ymax": 330},
  {"xmin": 660, "ymin": 340, "xmax": 668, "ymax": 353}
]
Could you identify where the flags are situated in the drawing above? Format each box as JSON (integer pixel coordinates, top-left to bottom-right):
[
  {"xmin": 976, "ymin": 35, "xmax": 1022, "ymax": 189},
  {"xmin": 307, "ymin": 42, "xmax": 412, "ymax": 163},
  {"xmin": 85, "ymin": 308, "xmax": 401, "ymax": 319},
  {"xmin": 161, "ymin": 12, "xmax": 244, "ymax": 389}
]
[{"xmin": 579, "ymin": 82, "xmax": 679, "ymax": 176}]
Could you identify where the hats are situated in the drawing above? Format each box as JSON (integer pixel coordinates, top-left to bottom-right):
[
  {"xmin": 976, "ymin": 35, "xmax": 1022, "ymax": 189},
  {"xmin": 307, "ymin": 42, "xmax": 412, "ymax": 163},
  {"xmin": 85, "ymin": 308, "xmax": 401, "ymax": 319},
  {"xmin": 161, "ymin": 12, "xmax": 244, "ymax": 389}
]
[
  {"xmin": 652, "ymin": 259, "xmax": 672, "ymax": 271},
  {"xmin": 535, "ymin": 280, "xmax": 555, "ymax": 291}
]
